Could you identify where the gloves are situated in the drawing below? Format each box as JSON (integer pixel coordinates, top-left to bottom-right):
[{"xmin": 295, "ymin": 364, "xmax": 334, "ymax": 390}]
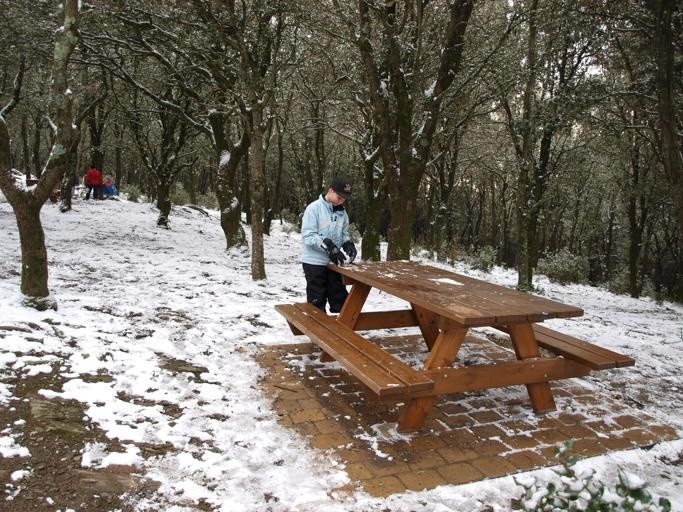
[
  {"xmin": 320, "ymin": 237, "xmax": 347, "ymax": 266},
  {"xmin": 343, "ymin": 241, "xmax": 357, "ymax": 264}
]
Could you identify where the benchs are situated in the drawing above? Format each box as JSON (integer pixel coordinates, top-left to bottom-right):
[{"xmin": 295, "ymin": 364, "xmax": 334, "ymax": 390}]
[
  {"xmin": 274, "ymin": 300, "xmax": 437, "ymax": 397},
  {"xmin": 489, "ymin": 319, "xmax": 635, "ymax": 375}
]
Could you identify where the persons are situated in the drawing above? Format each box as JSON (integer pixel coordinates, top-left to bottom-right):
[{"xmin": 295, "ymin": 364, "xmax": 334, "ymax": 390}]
[
  {"xmin": 101, "ymin": 176, "xmax": 120, "ymax": 202},
  {"xmin": 81, "ymin": 164, "xmax": 102, "ymax": 202},
  {"xmin": 295, "ymin": 176, "xmax": 358, "ymax": 316}
]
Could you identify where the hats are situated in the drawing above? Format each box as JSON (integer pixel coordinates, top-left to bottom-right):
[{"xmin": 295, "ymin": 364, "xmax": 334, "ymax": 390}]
[{"xmin": 330, "ymin": 177, "xmax": 356, "ymax": 200}]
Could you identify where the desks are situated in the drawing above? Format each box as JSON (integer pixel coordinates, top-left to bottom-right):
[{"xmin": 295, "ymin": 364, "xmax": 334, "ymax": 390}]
[{"xmin": 336, "ymin": 254, "xmax": 582, "ymax": 411}]
